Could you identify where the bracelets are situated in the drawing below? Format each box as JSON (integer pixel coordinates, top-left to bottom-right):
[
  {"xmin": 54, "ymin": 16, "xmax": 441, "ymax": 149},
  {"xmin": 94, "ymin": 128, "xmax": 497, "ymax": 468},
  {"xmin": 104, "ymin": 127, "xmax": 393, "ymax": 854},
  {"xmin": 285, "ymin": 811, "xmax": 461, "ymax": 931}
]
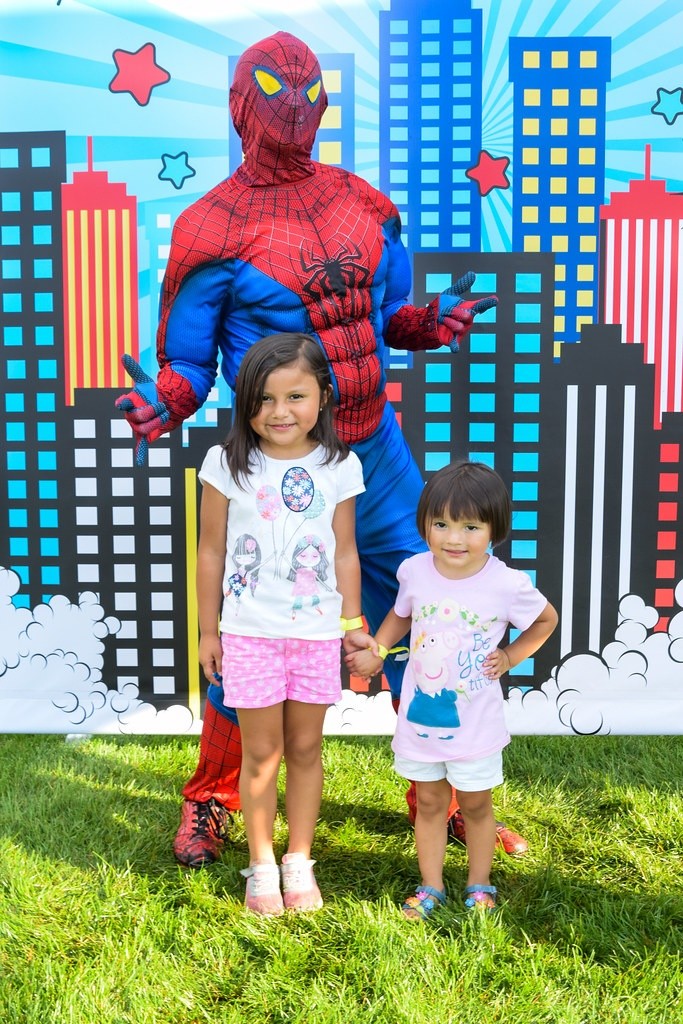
[
  {"xmin": 340, "ymin": 616, "xmax": 364, "ymax": 631},
  {"xmin": 368, "ymin": 643, "xmax": 410, "ymax": 660}
]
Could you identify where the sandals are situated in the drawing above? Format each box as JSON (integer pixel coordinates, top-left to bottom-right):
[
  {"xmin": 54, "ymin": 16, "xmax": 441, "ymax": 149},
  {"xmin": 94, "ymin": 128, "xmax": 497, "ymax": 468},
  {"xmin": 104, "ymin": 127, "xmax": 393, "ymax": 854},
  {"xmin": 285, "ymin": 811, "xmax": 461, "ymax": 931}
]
[
  {"xmin": 462, "ymin": 886, "xmax": 501, "ymax": 916},
  {"xmin": 402, "ymin": 886, "xmax": 448, "ymax": 922}
]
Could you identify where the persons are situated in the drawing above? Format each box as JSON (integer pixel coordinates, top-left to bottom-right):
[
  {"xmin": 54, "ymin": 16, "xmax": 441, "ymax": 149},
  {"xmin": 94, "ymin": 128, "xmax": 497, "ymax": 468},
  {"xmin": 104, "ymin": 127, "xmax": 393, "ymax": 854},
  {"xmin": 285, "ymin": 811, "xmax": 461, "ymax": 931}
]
[
  {"xmin": 345, "ymin": 462, "xmax": 559, "ymax": 920},
  {"xmin": 115, "ymin": 29, "xmax": 527, "ymax": 867},
  {"xmin": 199, "ymin": 333, "xmax": 380, "ymax": 917}
]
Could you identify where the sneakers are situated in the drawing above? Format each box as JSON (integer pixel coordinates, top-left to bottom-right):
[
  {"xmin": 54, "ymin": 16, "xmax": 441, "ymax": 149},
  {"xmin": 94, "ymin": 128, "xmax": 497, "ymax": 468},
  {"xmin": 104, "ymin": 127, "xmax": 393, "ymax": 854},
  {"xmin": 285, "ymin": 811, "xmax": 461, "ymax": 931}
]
[
  {"xmin": 239, "ymin": 865, "xmax": 283, "ymax": 919},
  {"xmin": 279, "ymin": 853, "xmax": 324, "ymax": 913},
  {"xmin": 174, "ymin": 797, "xmax": 234, "ymax": 869},
  {"xmin": 411, "ymin": 809, "xmax": 530, "ymax": 857}
]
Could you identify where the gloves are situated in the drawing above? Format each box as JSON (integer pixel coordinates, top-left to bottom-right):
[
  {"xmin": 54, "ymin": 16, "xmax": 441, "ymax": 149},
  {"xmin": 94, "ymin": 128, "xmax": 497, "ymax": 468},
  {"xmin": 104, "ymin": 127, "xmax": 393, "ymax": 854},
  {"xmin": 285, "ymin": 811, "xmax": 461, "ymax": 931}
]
[
  {"xmin": 113, "ymin": 355, "xmax": 173, "ymax": 465},
  {"xmin": 437, "ymin": 271, "xmax": 500, "ymax": 354}
]
